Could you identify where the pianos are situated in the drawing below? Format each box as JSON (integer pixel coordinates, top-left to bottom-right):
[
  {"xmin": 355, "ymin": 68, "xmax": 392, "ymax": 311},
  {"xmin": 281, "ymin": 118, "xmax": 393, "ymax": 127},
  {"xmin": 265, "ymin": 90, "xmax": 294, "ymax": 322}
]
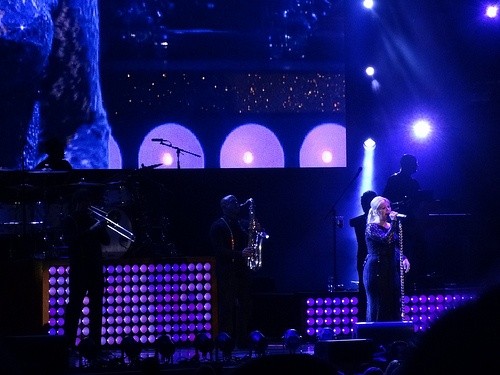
[{"xmin": 350, "ymin": 213, "xmax": 473, "ymax": 293}]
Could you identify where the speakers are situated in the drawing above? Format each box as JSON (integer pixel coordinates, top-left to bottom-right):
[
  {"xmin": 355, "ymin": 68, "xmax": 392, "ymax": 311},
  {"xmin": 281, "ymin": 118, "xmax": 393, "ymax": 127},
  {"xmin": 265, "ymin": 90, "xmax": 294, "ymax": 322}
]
[{"xmin": 316, "ymin": 320, "xmax": 415, "ymax": 364}]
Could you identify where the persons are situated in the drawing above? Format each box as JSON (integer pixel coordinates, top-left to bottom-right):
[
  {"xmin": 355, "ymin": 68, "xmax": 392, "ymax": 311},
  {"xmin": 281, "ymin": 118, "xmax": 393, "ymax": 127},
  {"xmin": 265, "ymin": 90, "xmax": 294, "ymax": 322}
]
[
  {"xmin": 363, "ymin": 196, "xmax": 411, "ymax": 322},
  {"xmin": 32, "ymin": 137, "xmax": 72, "ymax": 170},
  {"xmin": 62, "ymin": 188, "xmax": 110, "ymax": 352},
  {"xmin": 355, "ymin": 191, "xmax": 377, "ymax": 322},
  {"xmin": 384, "ymin": 153, "xmax": 426, "ymax": 262},
  {"xmin": 210, "ymin": 194, "xmax": 262, "ymax": 349}
]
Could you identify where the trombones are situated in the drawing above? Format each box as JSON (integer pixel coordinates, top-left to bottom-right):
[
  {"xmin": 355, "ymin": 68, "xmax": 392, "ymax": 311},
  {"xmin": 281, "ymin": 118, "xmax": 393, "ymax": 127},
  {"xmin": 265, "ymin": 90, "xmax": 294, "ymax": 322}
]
[{"xmin": 87, "ymin": 205, "xmax": 137, "ymax": 245}]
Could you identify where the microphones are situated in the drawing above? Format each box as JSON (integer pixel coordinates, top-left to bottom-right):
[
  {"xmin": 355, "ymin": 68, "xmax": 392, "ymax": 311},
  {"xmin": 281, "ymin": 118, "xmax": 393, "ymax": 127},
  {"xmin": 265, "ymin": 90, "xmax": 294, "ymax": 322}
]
[
  {"xmin": 151, "ymin": 138, "xmax": 167, "ymax": 141},
  {"xmin": 395, "ymin": 214, "xmax": 407, "ymax": 219}
]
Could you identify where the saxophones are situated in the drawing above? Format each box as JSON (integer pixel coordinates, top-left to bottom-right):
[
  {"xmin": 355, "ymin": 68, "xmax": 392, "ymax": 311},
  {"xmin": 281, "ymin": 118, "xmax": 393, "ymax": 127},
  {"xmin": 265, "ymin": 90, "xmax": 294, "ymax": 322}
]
[{"xmin": 240, "ymin": 198, "xmax": 269, "ymax": 273}]
